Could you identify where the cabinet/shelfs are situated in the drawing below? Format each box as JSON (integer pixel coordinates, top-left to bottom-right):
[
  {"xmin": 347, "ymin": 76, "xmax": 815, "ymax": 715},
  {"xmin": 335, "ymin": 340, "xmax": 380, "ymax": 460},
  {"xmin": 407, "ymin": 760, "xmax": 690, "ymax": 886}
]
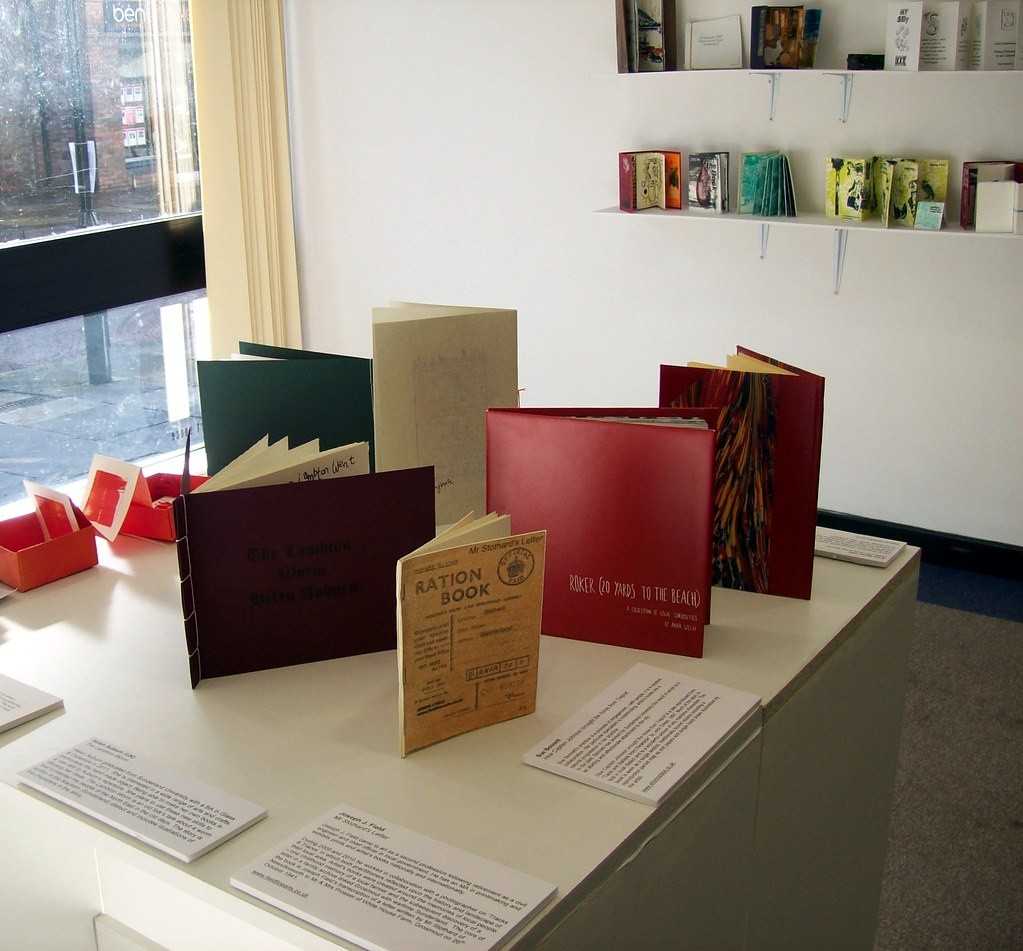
[{"xmin": 593, "ymin": 67, "xmax": 1023, "ymax": 296}]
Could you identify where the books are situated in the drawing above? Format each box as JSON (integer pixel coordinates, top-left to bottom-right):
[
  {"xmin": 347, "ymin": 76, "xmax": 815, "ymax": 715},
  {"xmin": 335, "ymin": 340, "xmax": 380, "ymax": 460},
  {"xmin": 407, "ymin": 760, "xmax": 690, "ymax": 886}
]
[
  {"xmin": 615, "ymin": 148, "xmax": 1023, "ymax": 236},
  {"xmin": 615, "ymin": 0, "xmax": 1023, "ymax": 73},
  {"xmin": 171, "ymin": 301, "xmax": 827, "ymax": 760}
]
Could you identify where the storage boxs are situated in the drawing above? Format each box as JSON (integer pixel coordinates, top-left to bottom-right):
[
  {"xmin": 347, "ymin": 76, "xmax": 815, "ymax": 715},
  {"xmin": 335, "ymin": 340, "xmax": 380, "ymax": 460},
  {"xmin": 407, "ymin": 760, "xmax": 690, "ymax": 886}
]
[
  {"xmin": 120, "ymin": 472, "xmax": 210, "ymax": 542},
  {"xmin": 0, "ymin": 512, "xmax": 99, "ymax": 592}
]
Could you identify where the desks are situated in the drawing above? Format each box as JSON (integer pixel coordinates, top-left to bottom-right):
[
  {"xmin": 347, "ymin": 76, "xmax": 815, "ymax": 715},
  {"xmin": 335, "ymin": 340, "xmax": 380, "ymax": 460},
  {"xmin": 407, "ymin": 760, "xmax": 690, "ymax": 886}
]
[{"xmin": 0, "ymin": 444, "xmax": 924, "ymax": 951}]
